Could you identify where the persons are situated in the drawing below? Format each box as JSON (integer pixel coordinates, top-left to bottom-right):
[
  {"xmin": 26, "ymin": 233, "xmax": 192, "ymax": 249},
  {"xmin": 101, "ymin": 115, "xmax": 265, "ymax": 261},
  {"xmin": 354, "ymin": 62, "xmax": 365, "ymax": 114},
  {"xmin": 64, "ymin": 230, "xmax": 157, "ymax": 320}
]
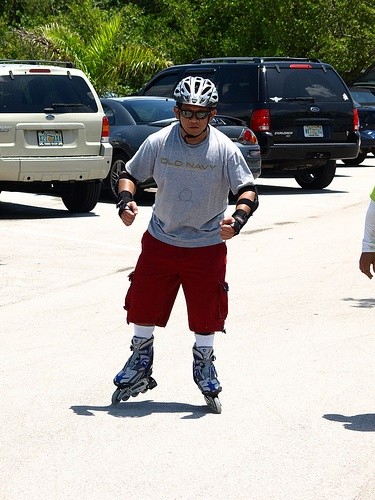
[
  {"xmin": 112, "ymin": 76, "xmax": 259, "ymax": 414},
  {"xmin": 359, "ymin": 186, "xmax": 375, "ymax": 279}
]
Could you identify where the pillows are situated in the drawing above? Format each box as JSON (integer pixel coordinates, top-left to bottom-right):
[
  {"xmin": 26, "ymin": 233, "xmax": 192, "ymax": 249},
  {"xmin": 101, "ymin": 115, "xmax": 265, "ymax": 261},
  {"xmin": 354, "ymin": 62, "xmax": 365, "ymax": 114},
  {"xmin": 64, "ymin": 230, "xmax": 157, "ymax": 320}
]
[{"xmin": 305, "ymin": 84, "xmax": 336, "ymax": 98}]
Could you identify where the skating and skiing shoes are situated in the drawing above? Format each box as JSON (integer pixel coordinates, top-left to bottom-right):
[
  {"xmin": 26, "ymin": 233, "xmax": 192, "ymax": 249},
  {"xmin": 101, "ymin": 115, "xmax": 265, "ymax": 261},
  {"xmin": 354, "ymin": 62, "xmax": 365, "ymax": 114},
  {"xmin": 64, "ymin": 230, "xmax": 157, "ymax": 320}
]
[
  {"xmin": 191, "ymin": 342, "xmax": 223, "ymax": 414},
  {"xmin": 111, "ymin": 335, "xmax": 158, "ymax": 405}
]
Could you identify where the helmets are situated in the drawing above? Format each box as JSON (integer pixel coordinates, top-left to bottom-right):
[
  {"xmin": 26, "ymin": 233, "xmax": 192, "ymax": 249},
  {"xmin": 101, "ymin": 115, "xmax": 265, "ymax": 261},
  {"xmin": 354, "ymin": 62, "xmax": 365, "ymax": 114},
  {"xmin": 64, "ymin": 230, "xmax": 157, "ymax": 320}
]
[{"xmin": 172, "ymin": 76, "xmax": 219, "ymax": 109}]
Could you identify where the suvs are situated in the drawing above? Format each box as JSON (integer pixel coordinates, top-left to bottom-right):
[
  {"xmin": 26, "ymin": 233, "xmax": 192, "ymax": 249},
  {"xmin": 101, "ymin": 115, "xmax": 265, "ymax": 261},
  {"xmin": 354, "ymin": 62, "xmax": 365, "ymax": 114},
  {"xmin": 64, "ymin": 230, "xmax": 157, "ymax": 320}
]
[
  {"xmin": 117, "ymin": 56, "xmax": 362, "ymax": 189},
  {"xmin": 0, "ymin": 58, "xmax": 114, "ymax": 213}
]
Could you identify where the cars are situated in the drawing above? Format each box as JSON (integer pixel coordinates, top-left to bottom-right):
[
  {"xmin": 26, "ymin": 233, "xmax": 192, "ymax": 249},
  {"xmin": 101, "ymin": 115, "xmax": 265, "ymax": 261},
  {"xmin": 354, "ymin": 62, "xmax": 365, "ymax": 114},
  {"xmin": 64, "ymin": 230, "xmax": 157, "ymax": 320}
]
[
  {"xmin": 99, "ymin": 95, "xmax": 262, "ymax": 206},
  {"xmin": 341, "ymin": 79, "xmax": 375, "ymax": 166}
]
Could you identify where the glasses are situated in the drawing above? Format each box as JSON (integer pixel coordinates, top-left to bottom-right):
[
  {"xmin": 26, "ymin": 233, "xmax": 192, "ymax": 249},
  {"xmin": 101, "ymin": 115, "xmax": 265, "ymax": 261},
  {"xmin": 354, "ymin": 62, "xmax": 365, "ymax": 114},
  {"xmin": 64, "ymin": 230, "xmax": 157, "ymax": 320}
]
[{"xmin": 177, "ymin": 107, "xmax": 211, "ymax": 120}]
